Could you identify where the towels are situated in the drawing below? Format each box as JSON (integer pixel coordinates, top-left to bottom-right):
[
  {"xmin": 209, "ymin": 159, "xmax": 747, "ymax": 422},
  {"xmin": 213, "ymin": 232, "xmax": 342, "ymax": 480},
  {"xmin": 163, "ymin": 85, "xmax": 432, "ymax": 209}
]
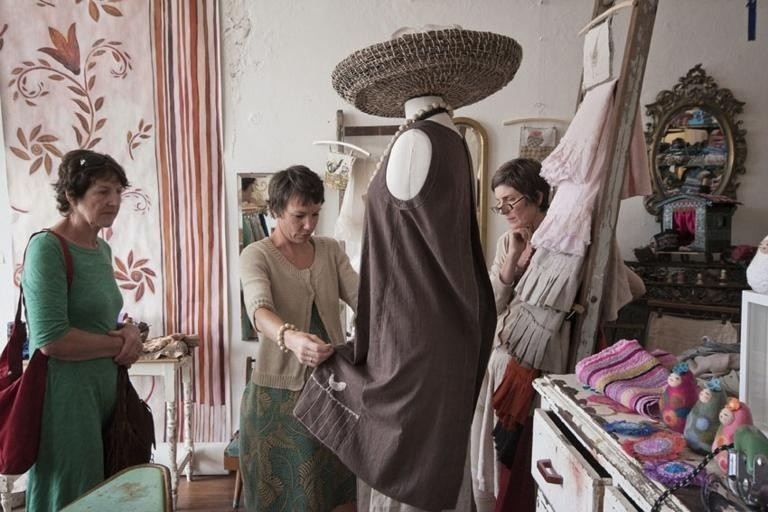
[{"xmin": 574, "ymin": 337, "xmax": 680, "ymax": 422}]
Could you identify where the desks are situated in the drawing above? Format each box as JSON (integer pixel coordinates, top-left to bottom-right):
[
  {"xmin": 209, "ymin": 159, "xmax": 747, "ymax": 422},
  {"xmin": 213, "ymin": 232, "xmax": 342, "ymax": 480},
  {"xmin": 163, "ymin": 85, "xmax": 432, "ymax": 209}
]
[
  {"xmin": 595, "ymin": 245, "xmax": 758, "ymax": 354},
  {"xmin": 530, "ymin": 375, "xmax": 767, "ymax": 512},
  {"xmin": 0, "ymin": 349, "xmax": 196, "ymax": 512}
]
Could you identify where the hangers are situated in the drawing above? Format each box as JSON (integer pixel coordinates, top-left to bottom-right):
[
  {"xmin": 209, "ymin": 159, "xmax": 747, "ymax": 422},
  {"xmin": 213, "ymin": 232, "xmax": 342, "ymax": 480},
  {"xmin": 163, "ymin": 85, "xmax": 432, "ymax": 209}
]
[
  {"xmin": 502, "ymin": 101, "xmax": 571, "ymax": 127},
  {"xmin": 576, "ymin": 0, "xmax": 636, "ymax": 37},
  {"xmin": 311, "ymin": 117, "xmax": 372, "ymax": 160}
]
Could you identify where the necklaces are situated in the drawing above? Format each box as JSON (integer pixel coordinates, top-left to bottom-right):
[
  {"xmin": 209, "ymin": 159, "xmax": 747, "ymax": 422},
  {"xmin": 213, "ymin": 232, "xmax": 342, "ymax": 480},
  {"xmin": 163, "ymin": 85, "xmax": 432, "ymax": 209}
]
[{"xmin": 359, "ymin": 100, "xmax": 457, "ymax": 204}]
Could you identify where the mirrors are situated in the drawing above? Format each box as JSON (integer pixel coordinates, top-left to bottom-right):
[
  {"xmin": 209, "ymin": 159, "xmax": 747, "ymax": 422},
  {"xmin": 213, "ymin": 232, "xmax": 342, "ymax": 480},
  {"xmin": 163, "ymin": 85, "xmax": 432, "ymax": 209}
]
[
  {"xmin": 453, "ymin": 117, "xmax": 488, "ymax": 266},
  {"xmin": 238, "ymin": 173, "xmax": 298, "ymax": 341},
  {"xmin": 644, "ymin": 63, "xmax": 748, "ymax": 212}
]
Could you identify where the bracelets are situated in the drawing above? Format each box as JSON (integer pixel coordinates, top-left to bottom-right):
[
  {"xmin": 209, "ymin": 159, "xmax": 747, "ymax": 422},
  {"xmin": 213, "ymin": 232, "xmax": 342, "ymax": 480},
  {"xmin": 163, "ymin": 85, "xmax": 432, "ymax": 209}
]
[{"xmin": 275, "ymin": 322, "xmax": 298, "ymax": 355}]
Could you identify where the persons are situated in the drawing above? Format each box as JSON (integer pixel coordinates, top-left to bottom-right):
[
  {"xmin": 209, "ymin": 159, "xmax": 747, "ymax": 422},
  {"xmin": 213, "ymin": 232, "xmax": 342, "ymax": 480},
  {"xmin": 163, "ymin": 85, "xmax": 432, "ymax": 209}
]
[
  {"xmin": 20, "ymin": 148, "xmax": 145, "ymax": 512},
  {"xmin": 470, "ymin": 156, "xmax": 649, "ymax": 512},
  {"xmin": 659, "ymin": 136, "xmax": 691, "ymax": 182},
  {"xmin": 237, "ymin": 163, "xmax": 360, "ymax": 512},
  {"xmin": 292, "ymin": 20, "xmax": 497, "ymax": 510},
  {"xmin": 241, "ymin": 176, "xmax": 258, "ymax": 340}
]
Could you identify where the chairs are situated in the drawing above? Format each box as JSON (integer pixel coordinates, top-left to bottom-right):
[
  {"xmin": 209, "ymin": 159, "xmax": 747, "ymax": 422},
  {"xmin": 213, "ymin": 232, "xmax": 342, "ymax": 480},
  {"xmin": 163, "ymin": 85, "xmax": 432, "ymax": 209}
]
[
  {"xmin": 58, "ymin": 464, "xmax": 170, "ymax": 512},
  {"xmin": 222, "ymin": 355, "xmax": 256, "ymax": 511}
]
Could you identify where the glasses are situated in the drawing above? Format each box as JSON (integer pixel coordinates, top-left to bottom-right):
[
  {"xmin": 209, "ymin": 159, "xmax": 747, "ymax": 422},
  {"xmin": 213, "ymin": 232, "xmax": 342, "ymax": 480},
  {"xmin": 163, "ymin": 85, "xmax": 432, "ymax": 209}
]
[{"xmin": 490, "ymin": 196, "xmax": 526, "ymax": 214}]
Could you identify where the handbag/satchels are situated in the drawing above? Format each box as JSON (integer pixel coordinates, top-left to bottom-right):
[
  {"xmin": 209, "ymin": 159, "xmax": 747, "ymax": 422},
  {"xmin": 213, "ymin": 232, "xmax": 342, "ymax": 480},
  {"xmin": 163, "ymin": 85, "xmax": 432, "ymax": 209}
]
[
  {"xmin": 728, "ymin": 424, "xmax": 768, "ymax": 512},
  {"xmin": 0, "ymin": 322, "xmax": 48, "ymax": 474},
  {"xmin": 105, "ymin": 366, "xmax": 155, "ymax": 475}
]
